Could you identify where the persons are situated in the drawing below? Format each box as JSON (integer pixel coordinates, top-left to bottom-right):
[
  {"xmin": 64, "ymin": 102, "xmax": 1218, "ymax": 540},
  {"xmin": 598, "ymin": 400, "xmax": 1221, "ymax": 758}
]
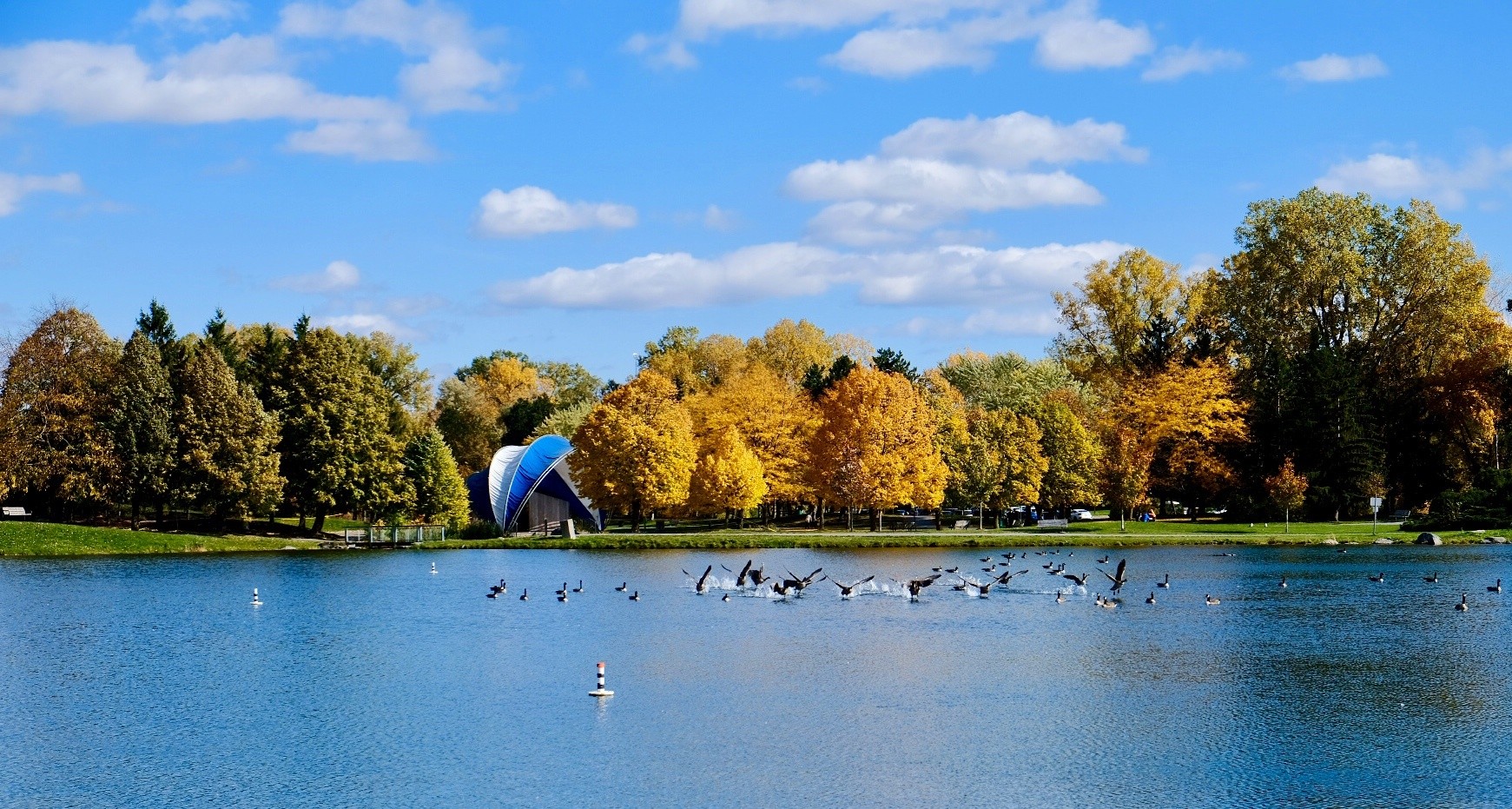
[
  {"xmin": 1136, "ymin": 509, "xmax": 1156, "ymax": 522},
  {"xmin": 1005, "ymin": 510, "xmax": 1017, "ymax": 528},
  {"xmin": 805, "ymin": 511, "xmax": 812, "ymax": 529}
]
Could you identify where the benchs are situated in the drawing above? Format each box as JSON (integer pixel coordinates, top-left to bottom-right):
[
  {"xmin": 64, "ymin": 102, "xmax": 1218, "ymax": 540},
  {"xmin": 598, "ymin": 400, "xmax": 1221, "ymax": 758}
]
[
  {"xmin": 1388, "ymin": 510, "xmax": 1411, "ymax": 522},
  {"xmin": 1037, "ymin": 519, "xmax": 1068, "ymax": 533},
  {"xmin": 948, "ymin": 520, "xmax": 971, "ymax": 530},
  {"xmin": 888, "ymin": 522, "xmax": 917, "ymax": 532},
  {"xmin": 1, "ymin": 506, "xmax": 32, "ymax": 521}
]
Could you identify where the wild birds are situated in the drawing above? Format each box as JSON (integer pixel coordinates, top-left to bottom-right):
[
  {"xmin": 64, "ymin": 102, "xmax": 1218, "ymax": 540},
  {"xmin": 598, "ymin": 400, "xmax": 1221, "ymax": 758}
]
[{"xmin": 478, "ymin": 543, "xmax": 1501, "ymax": 612}]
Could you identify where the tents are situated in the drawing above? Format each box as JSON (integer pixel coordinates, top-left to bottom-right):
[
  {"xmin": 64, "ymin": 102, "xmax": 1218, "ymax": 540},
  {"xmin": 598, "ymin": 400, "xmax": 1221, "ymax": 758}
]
[{"xmin": 465, "ymin": 434, "xmax": 612, "ymax": 537}]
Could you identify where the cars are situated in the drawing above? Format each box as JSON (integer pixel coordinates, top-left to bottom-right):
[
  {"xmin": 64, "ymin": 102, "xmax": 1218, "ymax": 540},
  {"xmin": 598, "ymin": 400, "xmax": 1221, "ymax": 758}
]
[
  {"xmin": 862, "ymin": 505, "xmax": 1093, "ymax": 521},
  {"xmin": 1136, "ymin": 508, "xmax": 1156, "ymax": 522}
]
